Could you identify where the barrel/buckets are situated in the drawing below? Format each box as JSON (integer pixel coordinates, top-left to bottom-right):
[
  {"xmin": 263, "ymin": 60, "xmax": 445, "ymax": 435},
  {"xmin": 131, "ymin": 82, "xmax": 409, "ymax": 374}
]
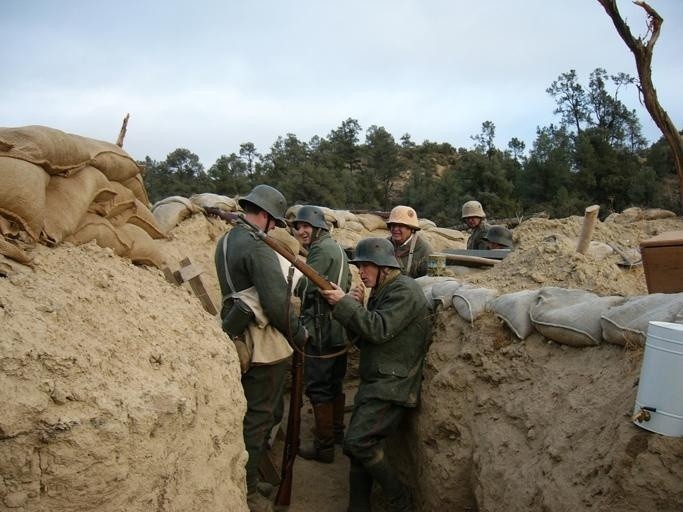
[{"xmin": 631, "ymin": 320, "xmax": 683, "ymax": 438}]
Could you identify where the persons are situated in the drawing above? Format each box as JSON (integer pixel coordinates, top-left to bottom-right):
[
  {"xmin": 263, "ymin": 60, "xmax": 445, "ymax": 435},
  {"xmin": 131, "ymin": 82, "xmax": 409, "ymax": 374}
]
[
  {"xmin": 461, "ymin": 200, "xmax": 490, "ymax": 250},
  {"xmin": 215, "ymin": 184, "xmax": 309, "ymax": 511},
  {"xmin": 386, "ymin": 205, "xmax": 430, "ymax": 280},
  {"xmin": 318, "ymin": 237, "xmax": 432, "ymax": 511},
  {"xmin": 289, "ymin": 206, "xmax": 352, "ymax": 463}
]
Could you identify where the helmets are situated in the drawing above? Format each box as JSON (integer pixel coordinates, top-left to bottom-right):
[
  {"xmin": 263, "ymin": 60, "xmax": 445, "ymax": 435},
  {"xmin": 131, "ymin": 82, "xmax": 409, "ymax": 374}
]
[
  {"xmin": 292, "ymin": 205, "xmax": 330, "ymax": 232},
  {"xmin": 461, "ymin": 201, "xmax": 487, "ymax": 220},
  {"xmin": 237, "ymin": 184, "xmax": 289, "ymax": 228},
  {"xmin": 348, "ymin": 237, "xmax": 403, "ymax": 268},
  {"xmin": 386, "ymin": 205, "xmax": 422, "ymax": 231}
]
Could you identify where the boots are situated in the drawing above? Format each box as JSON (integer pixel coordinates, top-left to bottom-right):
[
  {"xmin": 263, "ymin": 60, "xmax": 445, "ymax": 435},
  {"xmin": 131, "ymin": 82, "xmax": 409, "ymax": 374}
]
[
  {"xmin": 245, "ymin": 448, "xmax": 274, "ymax": 511},
  {"xmin": 334, "ymin": 393, "xmax": 346, "ymax": 445},
  {"xmin": 298, "ymin": 400, "xmax": 336, "ymax": 463}
]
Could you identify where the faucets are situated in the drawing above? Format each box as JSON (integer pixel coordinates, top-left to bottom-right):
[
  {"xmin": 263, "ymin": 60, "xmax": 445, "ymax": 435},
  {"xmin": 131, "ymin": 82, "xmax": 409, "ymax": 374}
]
[{"xmin": 632, "ymin": 406, "xmax": 656, "ymax": 422}]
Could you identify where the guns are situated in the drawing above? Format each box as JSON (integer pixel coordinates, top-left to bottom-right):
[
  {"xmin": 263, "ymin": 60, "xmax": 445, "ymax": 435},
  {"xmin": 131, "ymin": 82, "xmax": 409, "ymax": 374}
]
[
  {"xmin": 237, "ymin": 213, "xmax": 335, "ymax": 290},
  {"xmin": 274, "ymin": 308, "xmax": 303, "ymax": 510}
]
